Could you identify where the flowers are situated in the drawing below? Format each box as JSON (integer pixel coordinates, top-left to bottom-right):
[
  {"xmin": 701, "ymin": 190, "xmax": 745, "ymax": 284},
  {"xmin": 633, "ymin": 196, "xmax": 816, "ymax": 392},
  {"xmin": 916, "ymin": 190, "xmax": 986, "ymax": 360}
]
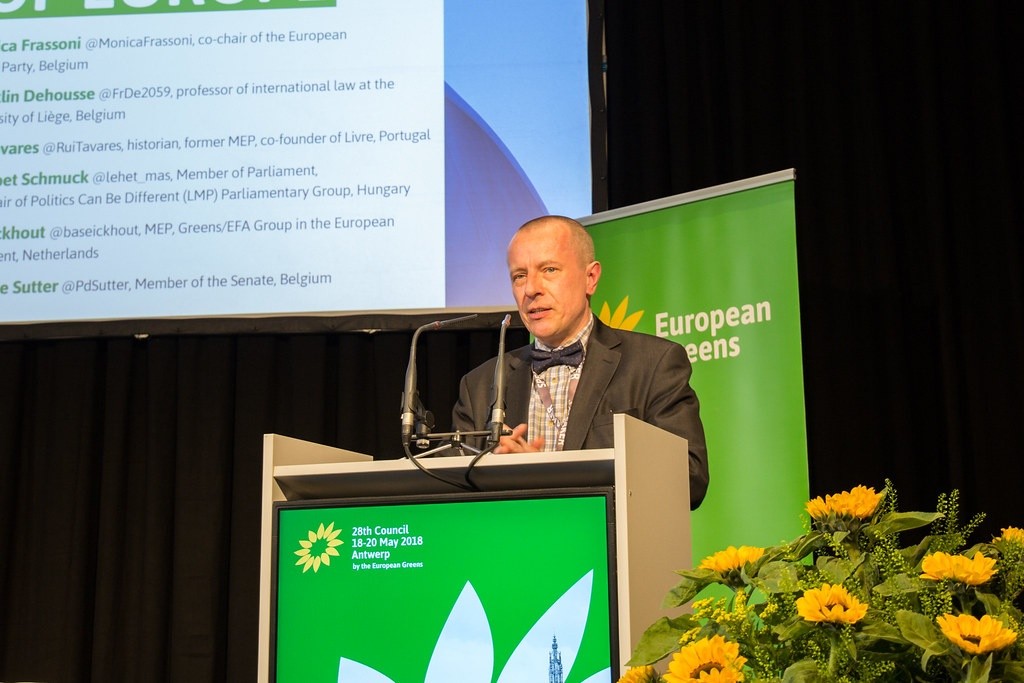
[{"xmin": 615, "ymin": 476, "xmax": 1024, "ymax": 683}]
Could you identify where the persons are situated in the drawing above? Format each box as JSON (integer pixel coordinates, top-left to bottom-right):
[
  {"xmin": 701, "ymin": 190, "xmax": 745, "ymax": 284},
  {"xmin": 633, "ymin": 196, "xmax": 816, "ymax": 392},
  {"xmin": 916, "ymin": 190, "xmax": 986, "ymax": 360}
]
[{"xmin": 436, "ymin": 215, "xmax": 709, "ymax": 511}]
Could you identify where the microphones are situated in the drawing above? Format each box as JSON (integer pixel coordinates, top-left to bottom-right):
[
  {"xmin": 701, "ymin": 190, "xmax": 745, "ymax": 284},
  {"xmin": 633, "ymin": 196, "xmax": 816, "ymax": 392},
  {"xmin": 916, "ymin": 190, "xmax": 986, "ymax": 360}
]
[
  {"xmin": 492, "ymin": 313, "xmax": 512, "ymax": 443},
  {"xmin": 401, "ymin": 313, "xmax": 479, "ymax": 447}
]
